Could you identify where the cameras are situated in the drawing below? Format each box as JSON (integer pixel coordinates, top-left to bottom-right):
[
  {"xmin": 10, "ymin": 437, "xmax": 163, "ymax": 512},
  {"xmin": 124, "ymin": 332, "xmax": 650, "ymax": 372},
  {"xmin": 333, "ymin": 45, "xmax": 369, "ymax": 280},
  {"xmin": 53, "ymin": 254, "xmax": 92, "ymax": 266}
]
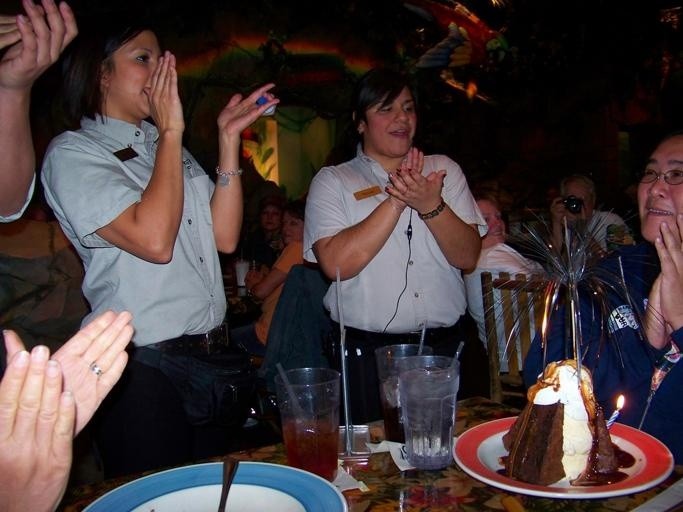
[{"xmin": 559, "ymin": 195, "xmax": 583, "ymax": 216}]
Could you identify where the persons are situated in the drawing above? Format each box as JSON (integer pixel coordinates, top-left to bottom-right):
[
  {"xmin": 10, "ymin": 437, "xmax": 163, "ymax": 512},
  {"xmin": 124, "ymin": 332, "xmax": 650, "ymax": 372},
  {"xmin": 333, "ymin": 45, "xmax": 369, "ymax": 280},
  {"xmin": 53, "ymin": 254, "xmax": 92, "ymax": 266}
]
[
  {"xmin": 254, "ymin": 194, "xmax": 290, "ymax": 262},
  {"xmin": 0, "ymin": 309, "xmax": 136, "ymax": 512},
  {"xmin": 463, "ymin": 181, "xmax": 547, "ymax": 392},
  {"xmin": 41, "ymin": 15, "xmax": 281, "ymax": 475},
  {"xmin": 549, "ymin": 175, "xmax": 629, "ymax": 270},
  {"xmin": 1, "ymin": 0, "xmax": 90, "ymax": 377},
  {"xmin": 521, "ymin": 133, "xmax": 683, "ymax": 466},
  {"xmin": 228, "ymin": 199, "xmax": 306, "ymax": 357},
  {"xmin": 303, "ymin": 67, "xmax": 490, "ymax": 426}
]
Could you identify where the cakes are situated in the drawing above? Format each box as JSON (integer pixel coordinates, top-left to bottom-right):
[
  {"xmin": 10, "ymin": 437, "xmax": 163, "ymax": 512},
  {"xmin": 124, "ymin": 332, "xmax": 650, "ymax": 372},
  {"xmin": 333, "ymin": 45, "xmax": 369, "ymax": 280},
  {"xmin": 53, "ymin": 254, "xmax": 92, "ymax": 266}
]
[{"xmin": 499, "ymin": 358, "xmax": 615, "ymax": 486}]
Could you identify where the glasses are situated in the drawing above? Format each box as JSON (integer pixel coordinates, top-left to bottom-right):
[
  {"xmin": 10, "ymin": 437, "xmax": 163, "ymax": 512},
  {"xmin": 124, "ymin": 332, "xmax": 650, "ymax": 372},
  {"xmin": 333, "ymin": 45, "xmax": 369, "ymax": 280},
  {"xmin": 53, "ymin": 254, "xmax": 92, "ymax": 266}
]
[
  {"xmin": 579, "ymin": 195, "xmax": 588, "ymax": 201},
  {"xmin": 636, "ymin": 168, "xmax": 683, "ymax": 188}
]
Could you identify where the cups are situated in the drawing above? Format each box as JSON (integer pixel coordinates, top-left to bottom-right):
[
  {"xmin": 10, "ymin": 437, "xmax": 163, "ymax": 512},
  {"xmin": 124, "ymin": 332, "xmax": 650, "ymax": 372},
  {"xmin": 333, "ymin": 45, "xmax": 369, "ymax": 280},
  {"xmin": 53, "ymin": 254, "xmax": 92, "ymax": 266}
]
[
  {"xmin": 376, "ymin": 344, "xmax": 432, "ymax": 443},
  {"xmin": 396, "ymin": 355, "xmax": 460, "ymax": 470},
  {"xmin": 274, "ymin": 366, "xmax": 341, "ymax": 482}
]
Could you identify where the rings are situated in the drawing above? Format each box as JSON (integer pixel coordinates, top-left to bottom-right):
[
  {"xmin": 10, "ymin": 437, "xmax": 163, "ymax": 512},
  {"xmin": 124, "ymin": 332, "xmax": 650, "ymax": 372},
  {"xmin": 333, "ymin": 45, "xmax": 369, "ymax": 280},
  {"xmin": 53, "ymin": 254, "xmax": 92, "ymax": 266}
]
[{"xmin": 87, "ymin": 363, "xmax": 106, "ymax": 380}]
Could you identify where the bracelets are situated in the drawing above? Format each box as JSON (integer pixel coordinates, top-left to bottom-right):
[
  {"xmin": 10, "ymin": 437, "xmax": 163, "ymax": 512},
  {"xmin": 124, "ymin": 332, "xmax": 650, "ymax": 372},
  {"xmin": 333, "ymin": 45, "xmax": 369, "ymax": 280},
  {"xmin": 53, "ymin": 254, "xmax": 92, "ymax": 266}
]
[
  {"xmin": 417, "ymin": 197, "xmax": 446, "ymax": 220},
  {"xmin": 215, "ymin": 165, "xmax": 244, "ymax": 179}
]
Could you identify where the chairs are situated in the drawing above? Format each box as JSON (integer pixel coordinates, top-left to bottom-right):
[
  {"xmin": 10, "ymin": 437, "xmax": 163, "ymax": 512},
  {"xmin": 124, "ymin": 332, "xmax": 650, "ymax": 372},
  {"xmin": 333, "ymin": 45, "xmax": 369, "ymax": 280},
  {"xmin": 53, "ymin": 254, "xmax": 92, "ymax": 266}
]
[{"xmin": 477, "ymin": 271, "xmax": 561, "ymax": 403}]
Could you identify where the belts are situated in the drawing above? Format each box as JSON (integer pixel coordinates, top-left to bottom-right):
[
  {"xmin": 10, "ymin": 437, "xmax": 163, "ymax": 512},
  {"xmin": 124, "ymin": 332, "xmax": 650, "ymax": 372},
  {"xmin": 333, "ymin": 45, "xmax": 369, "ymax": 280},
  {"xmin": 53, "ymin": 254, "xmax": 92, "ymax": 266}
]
[
  {"xmin": 147, "ymin": 321, "xmax": 234, "ymax": 359},
  {"xmin": 345, "ymin": 326, "xmax": 453, "ymax": 346}
]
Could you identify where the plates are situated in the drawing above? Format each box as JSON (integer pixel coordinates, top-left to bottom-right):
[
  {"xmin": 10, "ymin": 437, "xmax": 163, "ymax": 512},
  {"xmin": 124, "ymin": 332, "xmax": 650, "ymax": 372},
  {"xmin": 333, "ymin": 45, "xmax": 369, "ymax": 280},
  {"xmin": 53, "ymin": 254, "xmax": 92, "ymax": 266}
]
[
  {"xmin": 453, "ymin": 415, "xmax": 664, "ymax": 499},
  {"xmin": 79, "ymin": 461, "xmax": 350, "ymax": 512}
]
